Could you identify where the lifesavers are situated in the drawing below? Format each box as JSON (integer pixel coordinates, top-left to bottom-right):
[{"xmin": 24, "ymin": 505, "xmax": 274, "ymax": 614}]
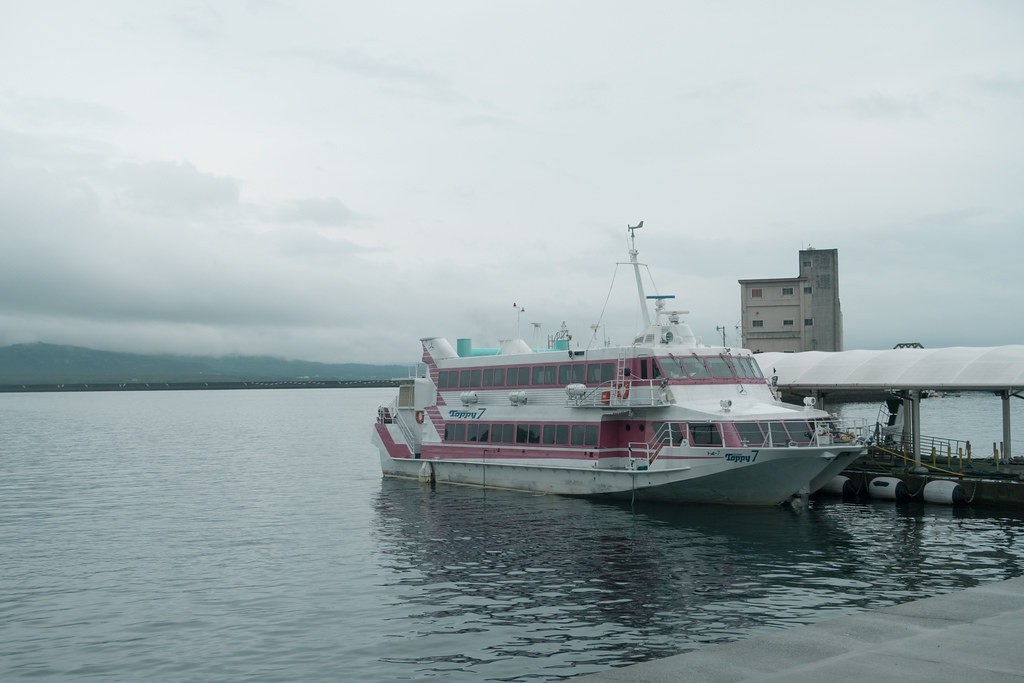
[
  {"xmin": 615, "ymin": 382, "xmax": 630, "ymax": 401},
  {"xmin": 414, "ymin": 410, "xmax": 425, "ymax": 425}
]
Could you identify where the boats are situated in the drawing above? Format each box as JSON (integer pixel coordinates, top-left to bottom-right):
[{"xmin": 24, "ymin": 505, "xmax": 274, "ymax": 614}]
[{"xmin": 369, "ymin": 219, "xmax": 869, "ymax": 507}]
